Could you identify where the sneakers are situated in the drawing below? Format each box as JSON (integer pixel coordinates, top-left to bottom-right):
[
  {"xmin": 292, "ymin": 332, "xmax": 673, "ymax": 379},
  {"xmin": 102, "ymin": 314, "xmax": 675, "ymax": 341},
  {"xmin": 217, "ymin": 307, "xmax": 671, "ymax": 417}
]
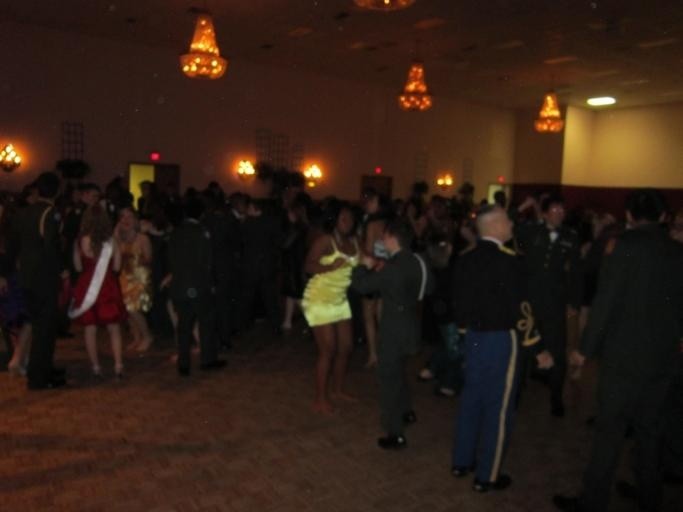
[{"xmin": 273, "ymin": 326, "xmax": 293, "ymax": 337}]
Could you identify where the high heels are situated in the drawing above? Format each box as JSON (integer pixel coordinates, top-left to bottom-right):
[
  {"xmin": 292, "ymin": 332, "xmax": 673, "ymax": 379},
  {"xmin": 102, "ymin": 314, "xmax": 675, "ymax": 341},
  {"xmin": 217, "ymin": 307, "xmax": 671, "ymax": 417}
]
[{"xmin": 7, "ymin": 341, "xmax": 226, "ymax": 391}]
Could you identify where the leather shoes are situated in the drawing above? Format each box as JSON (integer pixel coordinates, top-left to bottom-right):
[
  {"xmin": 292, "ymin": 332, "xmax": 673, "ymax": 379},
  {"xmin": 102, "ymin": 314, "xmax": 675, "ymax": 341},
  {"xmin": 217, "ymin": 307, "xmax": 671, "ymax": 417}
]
[{"xmin": 314, "ymin": 355, "xmax": 588, "ymax": 493}]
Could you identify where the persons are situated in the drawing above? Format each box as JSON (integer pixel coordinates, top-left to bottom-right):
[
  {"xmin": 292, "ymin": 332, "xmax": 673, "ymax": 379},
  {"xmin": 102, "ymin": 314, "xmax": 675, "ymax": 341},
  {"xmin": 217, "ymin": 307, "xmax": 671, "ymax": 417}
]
[
  {"xmin": 298, "ymin": 187, "xmax": 682, "ymax": 512},
  {"xmin": 1, "ymin": 168, "xmax": 322, "ymax": 393}
]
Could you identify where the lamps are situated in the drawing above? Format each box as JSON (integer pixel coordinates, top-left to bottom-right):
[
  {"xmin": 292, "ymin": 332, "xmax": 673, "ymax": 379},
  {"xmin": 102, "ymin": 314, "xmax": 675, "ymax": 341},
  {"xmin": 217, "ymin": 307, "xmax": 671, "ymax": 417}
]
[
  {"xmin": 531, "ymin": 88, "xmax": 566, "ymax": 135},
  {"xmin": 177, "ymin": 3, "xmax": 229, "ymax": 83},
  {"xmin": 396, "ymin": 54, "xmax": 432, "ymax": 114}
]
